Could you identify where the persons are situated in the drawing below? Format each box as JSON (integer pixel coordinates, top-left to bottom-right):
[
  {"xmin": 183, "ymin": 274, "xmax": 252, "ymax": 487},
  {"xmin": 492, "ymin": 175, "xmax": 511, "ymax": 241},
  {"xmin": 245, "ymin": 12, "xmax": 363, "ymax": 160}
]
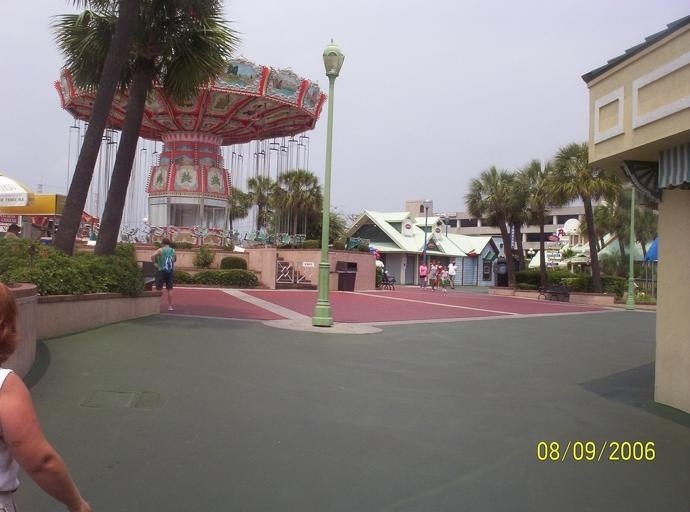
[
  {"xmin": 5, "ymin": 223, "xmax": 21, "ymax": 238},
  {"xmin": 0, "ymin": 282, "xmax": 93, "ymax": 510},
  {"xmin": 418, "ymin": 259, "xmax": 458, "ymax": 293},
  {"xmin": 150, "ymin": 238, "xmax": 177, "ymax": 311}
]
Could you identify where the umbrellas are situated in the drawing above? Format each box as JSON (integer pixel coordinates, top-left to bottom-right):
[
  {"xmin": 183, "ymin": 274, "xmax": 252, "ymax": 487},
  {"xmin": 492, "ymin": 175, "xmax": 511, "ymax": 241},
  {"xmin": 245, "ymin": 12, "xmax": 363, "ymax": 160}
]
[{"xmin": 0, "ymin": 172, "xmax": 36, "ymax": 208}]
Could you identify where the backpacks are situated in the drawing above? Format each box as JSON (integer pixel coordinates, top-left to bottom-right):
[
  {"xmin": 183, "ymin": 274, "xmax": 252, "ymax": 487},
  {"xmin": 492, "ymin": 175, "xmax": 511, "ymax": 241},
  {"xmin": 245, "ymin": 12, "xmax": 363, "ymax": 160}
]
[{"xmin": 162, "ymin": 248, "xmax": 174, "ymax": 274}]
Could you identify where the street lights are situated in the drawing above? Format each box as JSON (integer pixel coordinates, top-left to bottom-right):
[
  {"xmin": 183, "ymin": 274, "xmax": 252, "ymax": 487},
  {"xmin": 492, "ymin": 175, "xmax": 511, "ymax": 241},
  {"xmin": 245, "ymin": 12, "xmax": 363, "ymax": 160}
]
[
  {"xmin": 420, "ymin": 196, "xmax": 434, "ymax": 287},
  {"xmin": 445, "ymin": 214, "xmax": 450, "ymax": 238},
  {"xmin": 309, "ymin": 36, "xmax": 348, "ymax": 329},
  {"xmin": 526, "ymin": 247, "xmax": 536, "ymax": 271}
]
